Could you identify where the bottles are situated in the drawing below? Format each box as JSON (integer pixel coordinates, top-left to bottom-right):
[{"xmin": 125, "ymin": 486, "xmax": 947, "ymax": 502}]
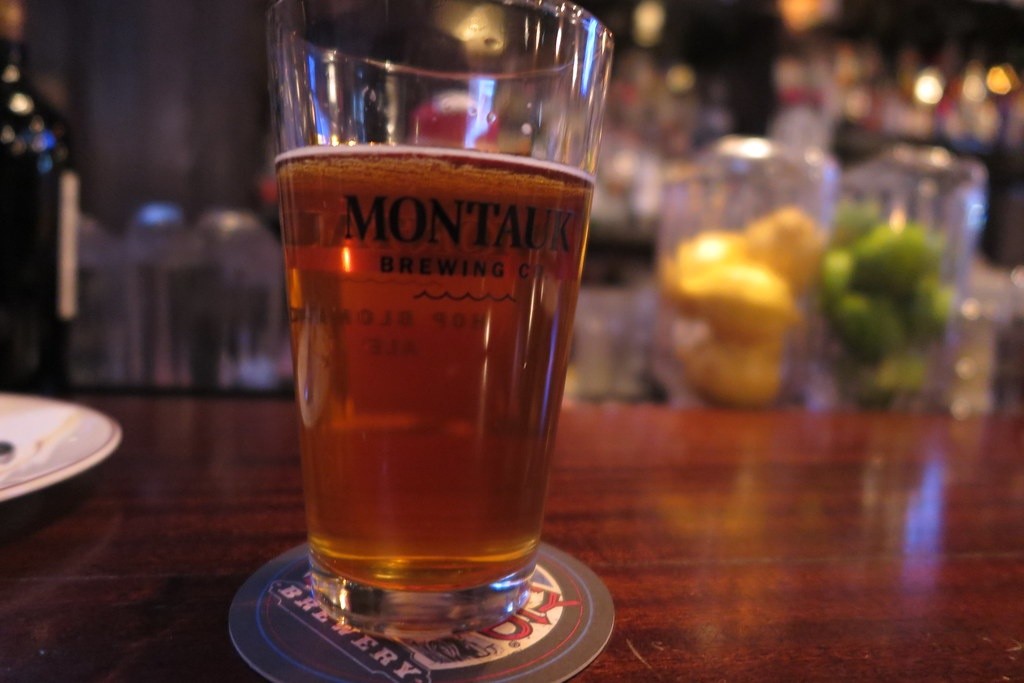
[
  {"xmin": 566, "ymin": 33, "xmax": 1023, "ymax": 245},
  {"xmin": 1, "ymin": 2, "xmax": 293, "ymax": 393}
]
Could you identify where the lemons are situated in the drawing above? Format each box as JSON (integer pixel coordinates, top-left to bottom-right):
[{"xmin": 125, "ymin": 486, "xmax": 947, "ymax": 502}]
[{"xmin": 664, "ymin": 200, "xmax": 959, "ymax": 411}]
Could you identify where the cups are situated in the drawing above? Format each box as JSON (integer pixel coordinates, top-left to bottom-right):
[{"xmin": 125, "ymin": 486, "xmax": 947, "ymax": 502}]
[
  {"xmin": 821, "ymin": 150, "xmax": 986, "ymax": 412},
  {"xmin": 653, "ymin": 151, "xmax": 839, "ymax": 412},
  {"xmin": 262, "ymin": 3, "xmax": 611, "ymax": 646}
]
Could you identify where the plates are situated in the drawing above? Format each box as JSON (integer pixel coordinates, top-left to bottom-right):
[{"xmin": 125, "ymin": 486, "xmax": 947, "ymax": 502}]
[{"xmin": 0, "ymin": 392, "xmax": 122, "ymax": 503}]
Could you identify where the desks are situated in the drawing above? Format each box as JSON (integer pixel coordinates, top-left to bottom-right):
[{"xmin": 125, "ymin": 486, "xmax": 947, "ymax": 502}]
[{"xmin": 0, "ymin": 393, "xmax": 1024, "ymax": 683}]
